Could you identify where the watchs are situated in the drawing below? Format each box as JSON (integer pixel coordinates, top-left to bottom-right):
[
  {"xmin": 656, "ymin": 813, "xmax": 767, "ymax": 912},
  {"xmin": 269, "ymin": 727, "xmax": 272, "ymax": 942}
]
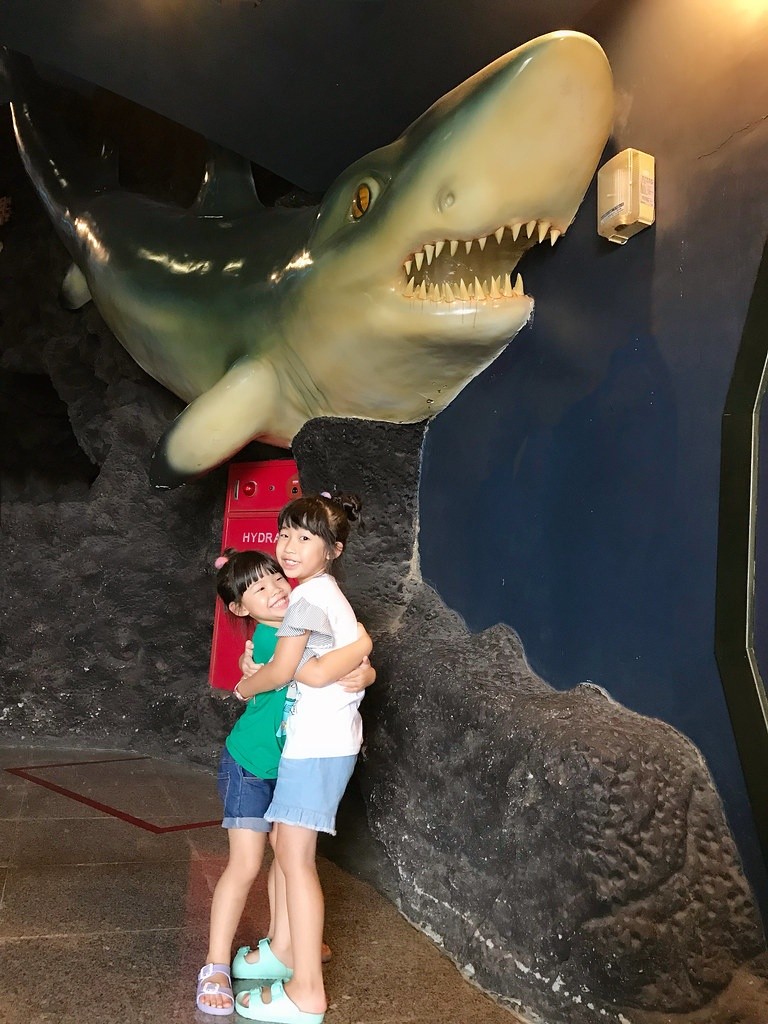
[{"xmin": 232, "ymin": 680, "xmax": 251, "ymax": 702}]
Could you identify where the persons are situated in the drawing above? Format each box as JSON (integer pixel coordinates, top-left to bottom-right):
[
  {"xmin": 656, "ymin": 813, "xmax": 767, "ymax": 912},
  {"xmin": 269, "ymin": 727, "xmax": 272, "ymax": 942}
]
[{"xmin": 191, "ymin": 491, "xmax": 376, "ymax": 1024}]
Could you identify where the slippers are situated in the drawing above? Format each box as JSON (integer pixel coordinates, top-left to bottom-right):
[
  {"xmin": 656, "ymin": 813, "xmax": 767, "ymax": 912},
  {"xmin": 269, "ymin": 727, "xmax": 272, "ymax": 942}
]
[
  {"xmin": 231, "ymin": 938, "xmax": 294, "ymax": 979},
  {"xmin": 235, "ymin": 978, "xmax": 324, "ymax": 1024}
]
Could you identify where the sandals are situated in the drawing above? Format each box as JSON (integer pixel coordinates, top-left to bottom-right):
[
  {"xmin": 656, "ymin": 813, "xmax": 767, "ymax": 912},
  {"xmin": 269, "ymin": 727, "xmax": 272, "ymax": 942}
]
[{"xmin": 196, "ymin": 963, "xmax": 234, "ymax": 1015}]
